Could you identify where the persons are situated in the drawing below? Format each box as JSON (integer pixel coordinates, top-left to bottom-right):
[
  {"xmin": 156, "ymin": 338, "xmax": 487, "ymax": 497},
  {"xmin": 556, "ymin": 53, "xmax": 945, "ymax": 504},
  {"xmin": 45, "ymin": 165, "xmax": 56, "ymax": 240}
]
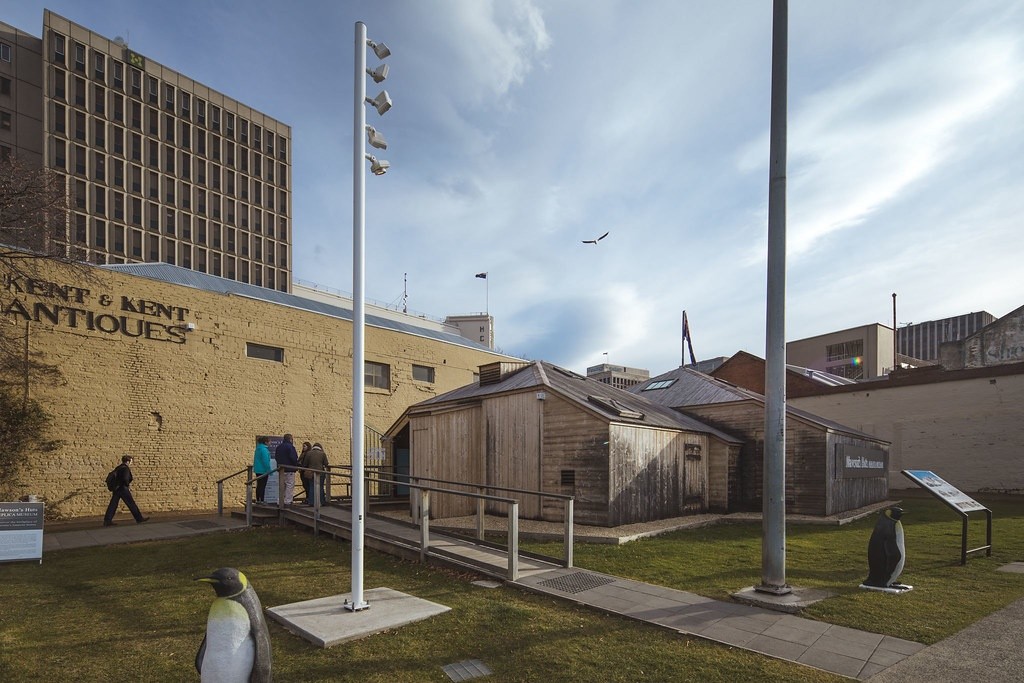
[
  {"xmin": 103, "ymin": 456, "xmax": 150, "ymax": 526},
  {"xmin": 276, "ymin": 434, "xmax": 330, "ymax": 506},
  {"xmin": 254, "ymin": 436, "xmax": 274, "ymax": 505}
]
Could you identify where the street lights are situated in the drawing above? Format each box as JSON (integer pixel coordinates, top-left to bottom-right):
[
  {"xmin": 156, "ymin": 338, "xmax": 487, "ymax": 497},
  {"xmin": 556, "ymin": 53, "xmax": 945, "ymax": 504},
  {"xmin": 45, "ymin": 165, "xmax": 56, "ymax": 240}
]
[{"xmin": 344, "ymin": 20, "xmax": 395, "ymax": 613}]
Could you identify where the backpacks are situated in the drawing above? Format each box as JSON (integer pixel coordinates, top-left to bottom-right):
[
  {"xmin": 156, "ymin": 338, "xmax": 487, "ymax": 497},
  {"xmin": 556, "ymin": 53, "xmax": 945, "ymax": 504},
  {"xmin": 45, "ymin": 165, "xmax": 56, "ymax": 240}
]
[{"xmin": 107, "ymin": 466, "xmax": 128, "ymax": 491}]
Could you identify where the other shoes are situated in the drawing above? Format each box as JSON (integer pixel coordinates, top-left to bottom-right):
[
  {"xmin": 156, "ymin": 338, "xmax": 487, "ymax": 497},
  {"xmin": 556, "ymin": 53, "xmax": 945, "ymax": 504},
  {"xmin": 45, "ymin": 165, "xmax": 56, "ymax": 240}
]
[
  {"xmin": 260, "ymin": 502, "xmax": 267, "ymax": 505},
  {"xmin": 321, "ymin": 502, "xmax": 326, "ymax": 506},
  {"xmin": 256, "ymin": 501, "xmax": 261, "ymax": 504},
  {"xmin": 305, "ymin": 503, "xmax": 314, "ymax": 507},
  {"xmin": 284, "ymin": 503, "xmax": 293, "ymax": 507},
  {"xmin": 136, "ymin": 517, "xmax": 149, "ymax": 523},
  {"xmin": 103, "ymin": 521, "xmax": 117, "ymax": 526},
  {"xmin": 300, "ymin": 500, "xmax": 308, "ymax": 504}
]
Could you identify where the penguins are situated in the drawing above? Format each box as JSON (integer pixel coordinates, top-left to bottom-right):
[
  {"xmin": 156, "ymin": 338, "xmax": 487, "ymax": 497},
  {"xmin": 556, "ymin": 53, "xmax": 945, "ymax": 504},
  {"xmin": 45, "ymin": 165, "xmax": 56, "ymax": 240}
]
[
  {"xmin": 862, "ymin": 506, "xmax": 911, "ymax": 590},
  {"xmin": 193, "ymin": 567, "xmax": 272, "ymax": 683}
]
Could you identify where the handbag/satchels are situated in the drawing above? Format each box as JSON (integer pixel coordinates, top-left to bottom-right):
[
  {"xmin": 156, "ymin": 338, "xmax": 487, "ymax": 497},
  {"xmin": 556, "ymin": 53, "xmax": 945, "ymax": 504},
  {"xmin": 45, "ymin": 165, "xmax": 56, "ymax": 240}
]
[{"xmin": 304, "ymin": 470, "xmax": 312, "ymax": 479}]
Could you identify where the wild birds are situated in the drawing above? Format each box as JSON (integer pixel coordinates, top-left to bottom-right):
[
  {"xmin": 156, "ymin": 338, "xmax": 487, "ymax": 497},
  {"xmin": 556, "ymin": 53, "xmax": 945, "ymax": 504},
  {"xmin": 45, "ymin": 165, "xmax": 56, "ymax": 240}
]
[{"xmin": 583, "ymin": 231, "xmax": 609, "ymax": 244}]
